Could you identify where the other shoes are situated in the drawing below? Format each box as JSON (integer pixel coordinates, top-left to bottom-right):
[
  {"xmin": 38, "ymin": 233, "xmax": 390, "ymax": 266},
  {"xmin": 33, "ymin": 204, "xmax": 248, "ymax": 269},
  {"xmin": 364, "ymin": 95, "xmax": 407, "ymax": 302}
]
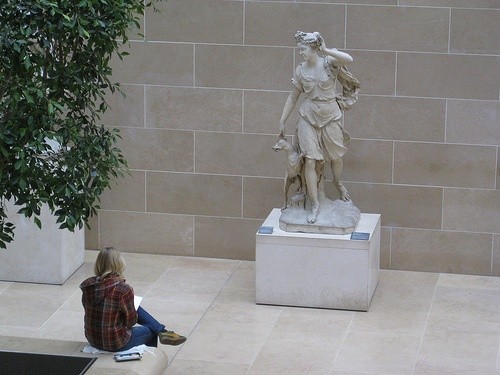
[{"xmin": 159, "ymin": 331, "xmax": 187, "ymax": 345}]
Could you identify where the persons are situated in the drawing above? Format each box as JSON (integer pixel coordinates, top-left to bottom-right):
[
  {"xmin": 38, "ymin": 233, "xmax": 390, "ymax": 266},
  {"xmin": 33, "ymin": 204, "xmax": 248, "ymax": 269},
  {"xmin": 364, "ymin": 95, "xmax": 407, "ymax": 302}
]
[
  {"xmin": 79, "ymin": 247, "xmax": 187, "ymax": 353},
  {"xmin": 276, "ymin": 29, "xmax": 360, "ymax": 225}
]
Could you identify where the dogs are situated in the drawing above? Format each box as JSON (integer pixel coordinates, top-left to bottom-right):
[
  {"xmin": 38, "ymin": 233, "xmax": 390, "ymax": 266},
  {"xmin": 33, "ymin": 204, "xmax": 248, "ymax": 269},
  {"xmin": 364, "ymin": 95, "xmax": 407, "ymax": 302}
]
[{"xmin": 272, "ymin": 139, "xmax": 307, "ymax": 211}]
